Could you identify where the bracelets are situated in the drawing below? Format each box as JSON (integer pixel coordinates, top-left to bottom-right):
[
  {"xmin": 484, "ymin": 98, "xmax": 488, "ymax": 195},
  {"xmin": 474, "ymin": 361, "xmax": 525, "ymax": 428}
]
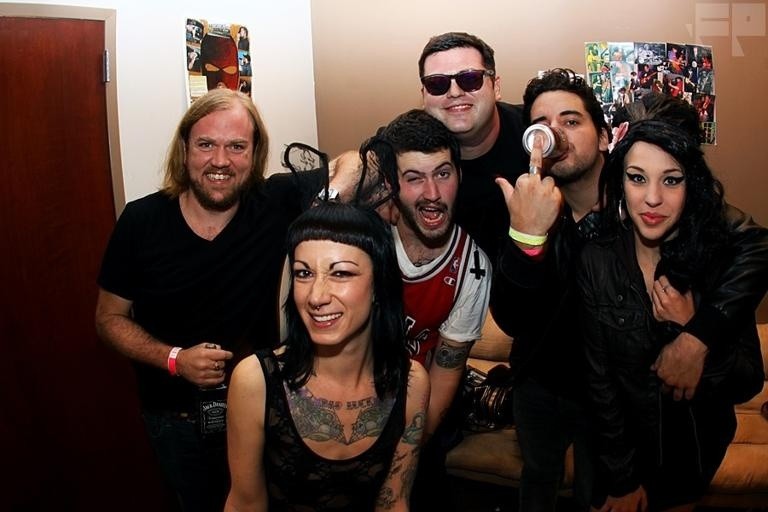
[
  {"xmin": 167, "ymin": 344, "xmax": 183, "ymax": 378},
  {"xmin": 507, "ymin": 225, "xmax": 549, "ymax": 246},
  {"xmin": 521, "ymin": 248, "xmax": 546, "ymax": 257}
]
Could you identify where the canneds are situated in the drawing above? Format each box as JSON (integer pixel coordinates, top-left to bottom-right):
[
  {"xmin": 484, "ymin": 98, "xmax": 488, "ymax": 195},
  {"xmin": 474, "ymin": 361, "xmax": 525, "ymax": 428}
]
[{"xmin": 522, "ymin": 122, "xmax": 568, "ymax": 159}]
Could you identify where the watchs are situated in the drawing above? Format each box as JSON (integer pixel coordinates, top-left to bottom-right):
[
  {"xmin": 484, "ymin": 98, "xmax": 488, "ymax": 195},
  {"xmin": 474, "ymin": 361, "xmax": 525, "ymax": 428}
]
[{"xmin": 311, "ymin": 187, "xmax": 343, "ymax": 206}]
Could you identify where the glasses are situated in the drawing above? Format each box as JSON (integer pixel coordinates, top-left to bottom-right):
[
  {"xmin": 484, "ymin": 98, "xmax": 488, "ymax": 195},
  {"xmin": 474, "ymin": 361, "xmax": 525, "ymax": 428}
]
[{"xmin": 421, "ymin": 69, "xmax": 495, "ymax": 97}]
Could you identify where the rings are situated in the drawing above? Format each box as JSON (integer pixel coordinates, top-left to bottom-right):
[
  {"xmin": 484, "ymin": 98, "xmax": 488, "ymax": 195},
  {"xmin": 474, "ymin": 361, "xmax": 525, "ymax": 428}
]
[
  {"xmin": 527, "ymin": 165, "xmax": 544, "ymax": 177},
  {"xmin": 661, "ymin": 283, "xmax": 671, "ymax": 291},
  {"xmin": 214, "ymin": 360, "xmax": 221, "ymax": 371}
]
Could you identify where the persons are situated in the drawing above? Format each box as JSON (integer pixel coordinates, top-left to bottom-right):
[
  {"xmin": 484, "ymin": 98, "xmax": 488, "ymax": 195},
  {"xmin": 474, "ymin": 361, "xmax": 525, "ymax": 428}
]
[
  {"xmin": 187, "ymin": 19, "xmax": 251, "ymax": 95},
  {"xmin": 561, "ymin": 94, "xmax": 768, "ymax": 511},
  {"xmin": 585, "ymin": 41, "xmax": 715, "ymax": 145},
  {"xmin": 415, "ymin": 35, "xmax": 535, "ymax": 263},
  {"xmin": 488, "ymin": 64, "xmax": 768, "ymax": 512},
  {"xmin": 94, "ymin": 87, "xmax": 378, "ymax": 512},
  {"xmin": 222, "ymin": 205, "xmax": 430, "ymax": 511},
  {"xmin": 355, "ymin": 108, "xmax": 494, "ymax": 448}
]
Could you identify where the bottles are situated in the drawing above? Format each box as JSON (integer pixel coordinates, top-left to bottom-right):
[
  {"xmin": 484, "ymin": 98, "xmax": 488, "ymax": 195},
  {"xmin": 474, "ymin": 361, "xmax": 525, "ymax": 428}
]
[{"xmin": 194, "ymin": 343, "xmax": 236, "ymax": 437}]
